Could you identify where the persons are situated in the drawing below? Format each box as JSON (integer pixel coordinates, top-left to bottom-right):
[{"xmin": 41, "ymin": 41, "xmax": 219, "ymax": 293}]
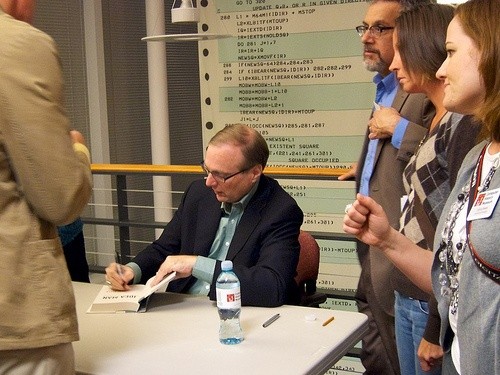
[
  {"xmin": 343, "ymin": 1, "xmax": 500, "ymax": 375},
  {"xmin": 337, "ymin": 1, "xmax": 437, "ymax": 375},
  {"xmin": 0, "ymin": 0, "xmax": 93, "ymax": 375},
  {"xmin": 104, "ymin": 123, "xmax": 303, "ymax": 308},
  {"xmin": 393, "ymin": 4, "xmax": 482, "ymax": 375}
]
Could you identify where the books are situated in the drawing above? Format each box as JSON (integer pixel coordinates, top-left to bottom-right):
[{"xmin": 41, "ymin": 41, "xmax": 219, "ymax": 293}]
[{"xmin": 85, "ymin": 271, "xmax": 177, "ymax": 314}]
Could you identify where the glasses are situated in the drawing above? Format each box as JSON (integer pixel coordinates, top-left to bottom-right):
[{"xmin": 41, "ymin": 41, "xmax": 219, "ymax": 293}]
[
  {"xmin": 200, "ymin": 161, "xmax": 255, "ymax": 182},
  {"xmin": 356, "ymin": 26, "xmax": 396, "ymax": 40}
]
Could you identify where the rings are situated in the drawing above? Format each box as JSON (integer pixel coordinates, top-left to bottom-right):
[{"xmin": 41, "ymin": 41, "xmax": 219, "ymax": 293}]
[
  {"xmin": 369, "ymin": 127, "xmax": 372, "ymax": 132},
  {"xmin": 106, "ymin": 280, "xmax": 111, "ymax": 285},
  {"xmin": 344, "ymin": 203, "xmax": 352, "ymax": 214}
]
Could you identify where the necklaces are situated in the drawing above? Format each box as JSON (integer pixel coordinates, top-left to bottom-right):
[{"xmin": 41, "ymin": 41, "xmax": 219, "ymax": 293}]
[{"xmin": 437, "ymin": 140, "xmax": 499, "ymax": 314}]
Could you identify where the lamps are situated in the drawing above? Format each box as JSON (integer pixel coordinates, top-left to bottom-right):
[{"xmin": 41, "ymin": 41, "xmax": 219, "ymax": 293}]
[{"xmin": 140, "ymin": 0, "xmax": 232, "ymax": 42}]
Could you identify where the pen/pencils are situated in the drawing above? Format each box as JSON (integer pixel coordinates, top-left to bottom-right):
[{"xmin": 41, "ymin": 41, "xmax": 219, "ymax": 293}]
[
  {"xmin": 262, "ymin": 313, "xmax": 280, "ymax": 328},
  {"xmin": 322, "ymin": 316, "xmax": 335, "ymax": 327},
  {"xmin": 114, "ymin": 250, "xmax": 127, "ymax": 291}
]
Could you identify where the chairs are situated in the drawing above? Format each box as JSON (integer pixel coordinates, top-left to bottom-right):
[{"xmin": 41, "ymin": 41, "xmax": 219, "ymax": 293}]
[{"xmin": 294, "ymin": 230, "xmax": 327, "ymax": 308}]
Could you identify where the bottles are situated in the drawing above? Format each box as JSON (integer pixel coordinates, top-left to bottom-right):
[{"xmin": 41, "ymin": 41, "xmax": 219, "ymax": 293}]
[{"xmin": 216, "ymin": 260, "xmax": 245, "ymax": 346}]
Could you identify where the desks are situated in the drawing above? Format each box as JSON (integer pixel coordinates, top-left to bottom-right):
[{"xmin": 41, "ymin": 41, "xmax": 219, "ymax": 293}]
[{"xmin": 70, "ymin": 280, "xmax": 370, "ymax": 375}]
